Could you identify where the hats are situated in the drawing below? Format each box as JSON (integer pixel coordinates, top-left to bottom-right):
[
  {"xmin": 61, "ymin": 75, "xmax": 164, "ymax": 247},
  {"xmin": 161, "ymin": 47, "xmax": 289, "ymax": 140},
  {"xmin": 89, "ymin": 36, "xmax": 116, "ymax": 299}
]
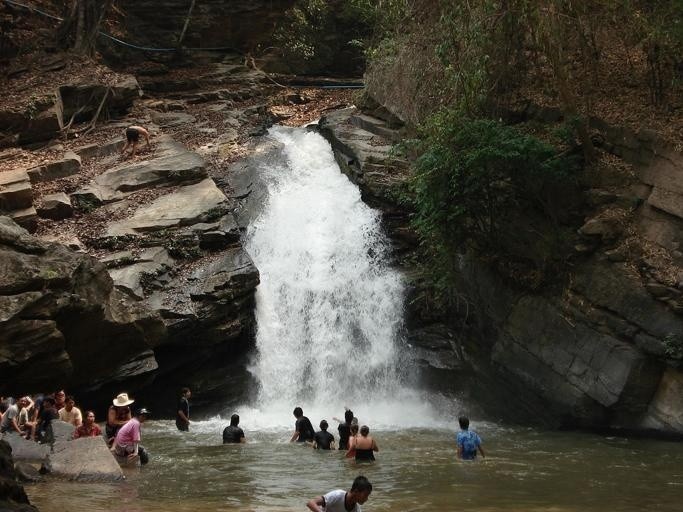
[
  {"xmin": 136, "ymin": 409, "xmax": 150, "ymax": 415},
  {"xmin": 113, "ymin": 393, "xmax": 134, "ymax": 407}
]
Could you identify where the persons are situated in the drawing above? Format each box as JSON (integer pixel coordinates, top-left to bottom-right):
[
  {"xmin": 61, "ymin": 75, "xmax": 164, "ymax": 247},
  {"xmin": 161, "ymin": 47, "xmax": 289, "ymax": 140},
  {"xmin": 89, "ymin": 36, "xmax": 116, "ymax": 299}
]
[
  {"xmin": 175, "ymin": 388, "xmax": 191, "ymax": 432},
  {"xmin": 105, "ymin": 392, "xmax": 148, "ymax": 465},
  {"xmin": 455, "ymin": 416, "xmax": 485, "ymax": 461},
  {"xmin": 0, "ymin": 390, "xmax": 102, "ymax": 446},
  {"xmin": 290, "ymin": 407, "xmax": 379, "ymax": 462},
  {"xmin": 306, "ymin": 476, "xmax": 372, "ymax": 512},
  {"xmin": 223, "ymin": 414, "xmax": 247, "ymax": 444}
]
